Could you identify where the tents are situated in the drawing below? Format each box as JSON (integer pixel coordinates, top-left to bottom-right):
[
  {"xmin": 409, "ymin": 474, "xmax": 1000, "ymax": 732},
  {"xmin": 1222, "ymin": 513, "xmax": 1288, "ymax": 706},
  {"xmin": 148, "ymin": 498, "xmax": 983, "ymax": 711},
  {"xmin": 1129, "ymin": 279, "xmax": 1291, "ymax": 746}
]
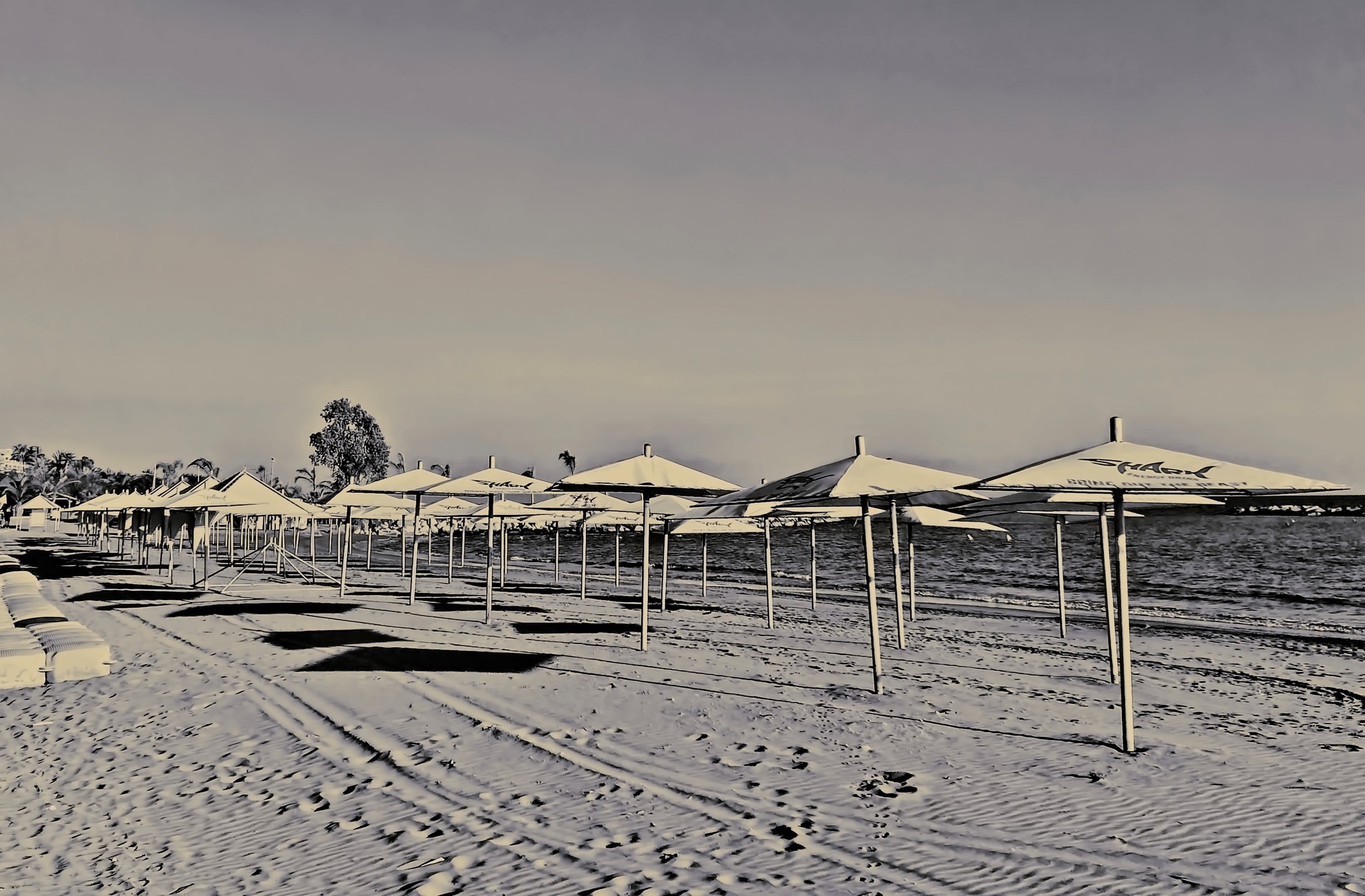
[
  {"xmin": 44, "ymin": 492, "xmax": 78, "ymax": 520},
  {"xmin": 0, "ymin": 493, "xmax": 21, "ymax": 527},
  {"xmin": 16, "ymin": 492, "xmax": 62, "ymax": 533}
]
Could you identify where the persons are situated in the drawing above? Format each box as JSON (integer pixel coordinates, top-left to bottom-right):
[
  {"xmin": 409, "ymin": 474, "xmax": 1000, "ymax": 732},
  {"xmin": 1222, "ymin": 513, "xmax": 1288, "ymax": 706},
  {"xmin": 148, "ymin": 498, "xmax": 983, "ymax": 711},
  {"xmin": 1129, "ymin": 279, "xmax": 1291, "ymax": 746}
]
[{"xmin": 4, "ymin": 504, "xmax": 13, "ymax": 529}]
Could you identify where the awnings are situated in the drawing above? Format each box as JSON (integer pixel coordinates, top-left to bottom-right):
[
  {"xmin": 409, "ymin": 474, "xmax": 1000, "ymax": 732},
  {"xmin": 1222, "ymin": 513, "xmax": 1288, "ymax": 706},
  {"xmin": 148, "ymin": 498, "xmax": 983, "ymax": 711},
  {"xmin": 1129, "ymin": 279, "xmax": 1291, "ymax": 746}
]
[{"xmin": 206, "ymin": 465, "xmax": 314, "ymax": 557}]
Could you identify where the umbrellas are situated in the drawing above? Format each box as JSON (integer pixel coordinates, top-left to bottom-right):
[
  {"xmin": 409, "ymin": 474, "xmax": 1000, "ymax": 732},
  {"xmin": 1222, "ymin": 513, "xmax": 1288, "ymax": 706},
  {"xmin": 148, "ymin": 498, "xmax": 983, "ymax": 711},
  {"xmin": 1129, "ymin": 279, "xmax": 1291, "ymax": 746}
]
[{"xmin": 55, "ymin": 416, "xmax": 1351, "ymax": 755}]
